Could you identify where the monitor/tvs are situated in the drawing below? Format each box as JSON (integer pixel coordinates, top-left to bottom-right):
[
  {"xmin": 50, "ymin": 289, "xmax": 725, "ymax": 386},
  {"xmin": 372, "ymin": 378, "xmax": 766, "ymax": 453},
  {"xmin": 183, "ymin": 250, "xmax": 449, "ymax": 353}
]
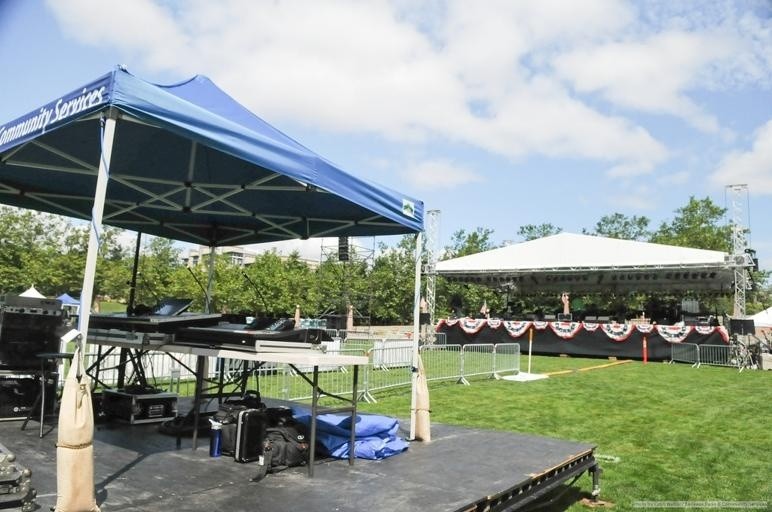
[{"xmin": 145, "ymin": 298, "xmax": 194, "ymax": 316}]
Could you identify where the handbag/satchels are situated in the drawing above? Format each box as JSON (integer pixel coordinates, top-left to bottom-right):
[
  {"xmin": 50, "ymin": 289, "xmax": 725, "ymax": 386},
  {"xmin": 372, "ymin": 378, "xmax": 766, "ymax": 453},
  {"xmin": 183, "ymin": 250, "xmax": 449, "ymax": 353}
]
[
  {"xmin": 215, "ymin": 389, "xmax": 263, "ymax": 456},
  {"xmin": 261, "ymin": 423, "xmax": 308, "ymax": 472},
  {"xmin": 235, "ymin": 407, "xmax": 293, "ymax": 463}
]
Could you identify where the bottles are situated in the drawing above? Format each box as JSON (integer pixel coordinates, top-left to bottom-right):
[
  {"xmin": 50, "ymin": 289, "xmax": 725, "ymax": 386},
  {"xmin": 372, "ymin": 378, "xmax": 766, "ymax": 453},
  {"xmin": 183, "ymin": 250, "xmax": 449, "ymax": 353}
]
[{"xmin": 209, "ymin": 418, "xmax": 223, "ymax": 457}]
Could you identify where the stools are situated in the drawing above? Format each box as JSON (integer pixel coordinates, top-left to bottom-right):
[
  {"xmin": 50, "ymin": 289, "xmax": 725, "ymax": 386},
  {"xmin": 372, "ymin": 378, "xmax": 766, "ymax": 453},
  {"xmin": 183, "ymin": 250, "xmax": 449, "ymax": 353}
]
[{"xmin": 22, "ymin": 351, "xmax": 71, "ymax": 438}]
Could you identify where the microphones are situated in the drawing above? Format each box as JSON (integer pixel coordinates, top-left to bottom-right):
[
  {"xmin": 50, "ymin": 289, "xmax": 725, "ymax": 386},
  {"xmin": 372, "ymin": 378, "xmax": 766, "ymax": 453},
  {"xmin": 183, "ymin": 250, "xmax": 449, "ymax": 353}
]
[
  {"xmin": 186, "ymin": 265, "xmax": 210, "ymax": 313},
  {"xmin": 137, "ymin": 270, "xmax": 166, "ymax": 316},
  {"xmin": 241, "ymin": 269, "xmax": 268, "ymax": 313}
]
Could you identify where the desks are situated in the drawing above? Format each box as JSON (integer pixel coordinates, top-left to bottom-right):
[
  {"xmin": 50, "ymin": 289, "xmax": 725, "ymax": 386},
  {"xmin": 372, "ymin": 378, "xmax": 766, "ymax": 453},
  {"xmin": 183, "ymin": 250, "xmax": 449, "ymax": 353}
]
[{"xmin": 48, "ymin": 335, "xmax": 369, "ymax": 478}]
